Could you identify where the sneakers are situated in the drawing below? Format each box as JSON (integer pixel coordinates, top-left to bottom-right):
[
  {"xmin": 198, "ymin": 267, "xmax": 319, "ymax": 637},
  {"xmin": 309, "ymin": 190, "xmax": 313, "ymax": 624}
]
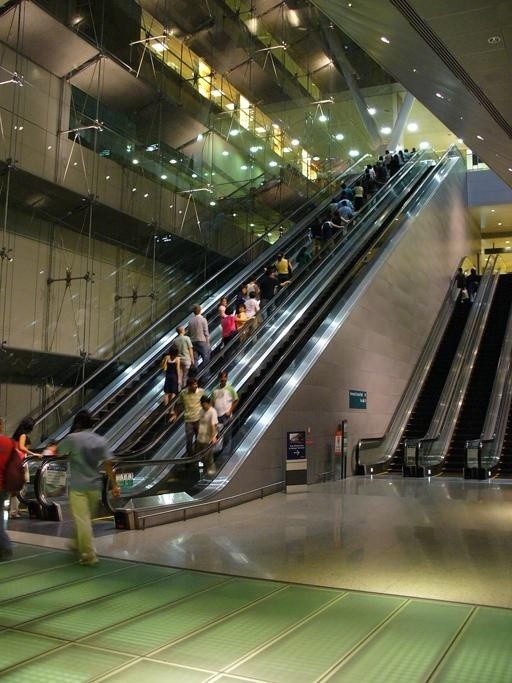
[
  {"xmin": 78, "ymin": 551, "xmax": 100, "ymax": 567},
  {"xmin": 9, "ymin": 509, "xmax": 26, "ymax": 519}
]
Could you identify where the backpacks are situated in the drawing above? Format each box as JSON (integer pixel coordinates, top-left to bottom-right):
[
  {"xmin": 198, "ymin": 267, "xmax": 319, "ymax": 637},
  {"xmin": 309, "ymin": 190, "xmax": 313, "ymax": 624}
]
[{"xmin": 2, "ymin": 436, "xmax": 26, "ymax": 493}]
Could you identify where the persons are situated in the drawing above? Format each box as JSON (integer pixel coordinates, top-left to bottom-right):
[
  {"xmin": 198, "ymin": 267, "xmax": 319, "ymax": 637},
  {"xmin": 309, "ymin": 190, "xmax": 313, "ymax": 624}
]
[
  {"xmin": 161, "ymin": 304, "xmax": 209, "ymax": 405},
  {"xmin": 311, "ymin": 181, "xmax": 364, "ymax": 261},
  {"xmin": 364, "ymin": 147, "xmax": 415, "ymax": 212},
  {"xmin": 168, "ymin": 371, "xmax": 239, "ymax": 473},
  {"xmin": 0, "ymin": 409, "xmax": 121, "ymax": 566},
  {"xmin": 456, "ymin": 266, "xmax": 479, "ymax": 305},
  {"xmin": 217, "ymin": 252, "xmax": 293, "ymax": 364},
  {"xmin": 296, "ymin": 246, "xmax": 312, "ymax": 277}
]
